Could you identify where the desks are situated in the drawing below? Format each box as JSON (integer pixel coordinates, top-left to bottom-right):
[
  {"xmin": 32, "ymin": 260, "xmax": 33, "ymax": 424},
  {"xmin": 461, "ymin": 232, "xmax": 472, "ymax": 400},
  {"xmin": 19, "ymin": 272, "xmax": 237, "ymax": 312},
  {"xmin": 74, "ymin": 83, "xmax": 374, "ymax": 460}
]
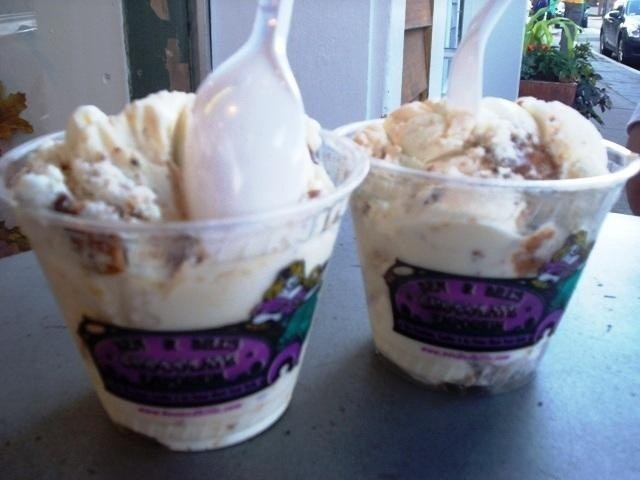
[{"xmin": 0, "ymin": 209, "xmax": 639, "ymax": 478}]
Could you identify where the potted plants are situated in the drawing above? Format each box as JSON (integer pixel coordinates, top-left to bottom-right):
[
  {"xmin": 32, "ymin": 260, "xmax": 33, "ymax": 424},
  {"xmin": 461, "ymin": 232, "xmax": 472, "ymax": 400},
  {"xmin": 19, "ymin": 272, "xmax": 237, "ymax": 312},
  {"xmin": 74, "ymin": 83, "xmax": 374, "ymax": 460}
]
[{"xmin": 517, "ymin": 6, "xmax": 611, "ymax": 126}]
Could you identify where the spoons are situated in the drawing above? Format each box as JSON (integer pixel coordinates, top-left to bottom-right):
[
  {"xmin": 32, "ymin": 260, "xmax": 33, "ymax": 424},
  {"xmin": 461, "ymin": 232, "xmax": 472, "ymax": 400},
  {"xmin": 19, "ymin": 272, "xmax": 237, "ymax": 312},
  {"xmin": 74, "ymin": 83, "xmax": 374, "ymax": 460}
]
[
  {"xmin": 446, "ymin": 0, "xmax": 514, "ymax": 101},
  {"xmin": 175, "ymin": 1, "xmax": 310, "ymax": 224}
]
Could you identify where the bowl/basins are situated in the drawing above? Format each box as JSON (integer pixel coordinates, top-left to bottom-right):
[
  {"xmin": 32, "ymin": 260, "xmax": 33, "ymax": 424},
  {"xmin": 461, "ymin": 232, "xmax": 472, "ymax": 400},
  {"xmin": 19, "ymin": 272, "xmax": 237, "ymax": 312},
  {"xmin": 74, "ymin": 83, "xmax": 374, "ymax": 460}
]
[
  {"xmin": 0, "ymin": 124, "xmax": 374, "ymax": 457},
  {"xmin": 329, "ymin": 120, "xmax": 639, "ymax": 393}
]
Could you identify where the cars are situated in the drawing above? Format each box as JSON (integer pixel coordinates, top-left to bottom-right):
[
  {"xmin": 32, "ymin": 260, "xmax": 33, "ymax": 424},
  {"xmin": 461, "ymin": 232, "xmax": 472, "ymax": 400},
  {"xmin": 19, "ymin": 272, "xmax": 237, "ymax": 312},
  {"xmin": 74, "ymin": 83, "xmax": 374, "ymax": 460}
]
[
  {"xmin": 554, "ymin": 0, "xmax": 590, "ymax": 28},
  {"xmin": 600, "ymin": 0, "xmax": 640, "ymax": 63}
]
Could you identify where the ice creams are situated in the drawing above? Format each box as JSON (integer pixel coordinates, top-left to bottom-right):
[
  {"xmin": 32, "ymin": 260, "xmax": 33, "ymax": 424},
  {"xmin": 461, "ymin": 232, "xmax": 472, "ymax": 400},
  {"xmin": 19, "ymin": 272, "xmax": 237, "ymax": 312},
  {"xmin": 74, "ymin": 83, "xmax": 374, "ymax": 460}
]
[
  {"xmin": 8, "ymin": 90, "xmax": 340, "ymax": 453},
  {"xmin": 340, "ymin": 97, "xmax": 613, "ymax": 387}
]
